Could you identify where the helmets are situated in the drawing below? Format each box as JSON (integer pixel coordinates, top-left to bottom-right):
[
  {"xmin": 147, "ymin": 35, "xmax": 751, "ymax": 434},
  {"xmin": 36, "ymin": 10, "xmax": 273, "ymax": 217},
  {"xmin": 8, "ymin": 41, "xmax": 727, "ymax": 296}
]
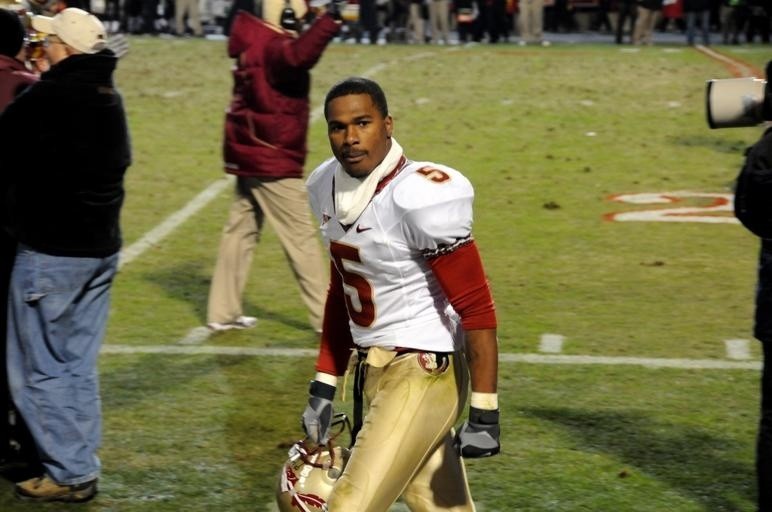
[{"xmin": 276, "ymin": 412, "xmax": 355, "ymax": 512}]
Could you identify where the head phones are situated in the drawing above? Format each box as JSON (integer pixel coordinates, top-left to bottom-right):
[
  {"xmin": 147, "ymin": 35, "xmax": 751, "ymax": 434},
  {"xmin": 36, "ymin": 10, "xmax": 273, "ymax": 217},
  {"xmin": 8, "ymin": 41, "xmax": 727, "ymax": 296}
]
[{"xmin": 281, "ymin": 8, "xmax": 298, "ymax": 28}]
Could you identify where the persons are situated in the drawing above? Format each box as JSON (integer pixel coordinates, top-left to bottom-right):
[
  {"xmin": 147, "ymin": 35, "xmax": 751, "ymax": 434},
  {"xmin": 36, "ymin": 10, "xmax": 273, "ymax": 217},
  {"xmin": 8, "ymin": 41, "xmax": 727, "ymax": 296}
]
[
  {"xmin": 297, "ymin": 76, "xmax": 502, "ymax": 511},
  {"xmin": 729, "ymin": 57, "xmax": 768, "ymax": 511},
  {"xmin": 1, "ymin": 1, "xmax": 259, "ymax": 38},
  {"xmin": 201, "ymin": 3, "xmax": 345, "ymax": 339},
  {"xmin": 516, "ymin": 0, "xmax": 771, "ymax": 50},
  {"xmin": 279, "ymin": 1, "xmax": 513, "ymax": 46}
]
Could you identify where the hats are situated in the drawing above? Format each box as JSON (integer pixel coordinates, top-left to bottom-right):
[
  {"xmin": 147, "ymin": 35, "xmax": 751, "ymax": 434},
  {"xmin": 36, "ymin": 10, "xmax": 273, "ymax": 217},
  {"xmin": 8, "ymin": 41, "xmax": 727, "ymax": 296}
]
[
  {"xmin": 31, "ymin": 7, "xmax": 108, "ymax": 53},
  {"xmin": 0, "ymin": 8, "xmax": 24, "ymax": 59},
  {"xmin": 263, "ymin": 0, "xmax": 308, "ymax": 28}
]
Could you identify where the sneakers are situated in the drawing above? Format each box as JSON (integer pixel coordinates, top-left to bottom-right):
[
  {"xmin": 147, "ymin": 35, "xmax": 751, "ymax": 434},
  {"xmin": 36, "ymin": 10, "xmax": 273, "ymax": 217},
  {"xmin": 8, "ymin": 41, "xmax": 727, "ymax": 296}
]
[
  {"xmin": 0, "ymin": 449, "xmax": 98, "ymax": 504},
  {"xmin": 207, "ymin": 316, "xmax": 258, "ymax": 331}
]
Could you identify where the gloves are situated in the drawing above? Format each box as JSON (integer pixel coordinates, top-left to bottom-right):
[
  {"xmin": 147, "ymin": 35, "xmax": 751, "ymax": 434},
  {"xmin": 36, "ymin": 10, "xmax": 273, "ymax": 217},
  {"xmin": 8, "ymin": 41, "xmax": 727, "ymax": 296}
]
[
  {"xmin": 302, "ymin": 379, "xmax": 337, "ymax": 448},
  {"xmin": 103, "ymin": 34, "xmax": 130, "ymax": 58},
  {"xmin": 450, "ymin": 391, "xmax": 501, "ymax": 458}
]
